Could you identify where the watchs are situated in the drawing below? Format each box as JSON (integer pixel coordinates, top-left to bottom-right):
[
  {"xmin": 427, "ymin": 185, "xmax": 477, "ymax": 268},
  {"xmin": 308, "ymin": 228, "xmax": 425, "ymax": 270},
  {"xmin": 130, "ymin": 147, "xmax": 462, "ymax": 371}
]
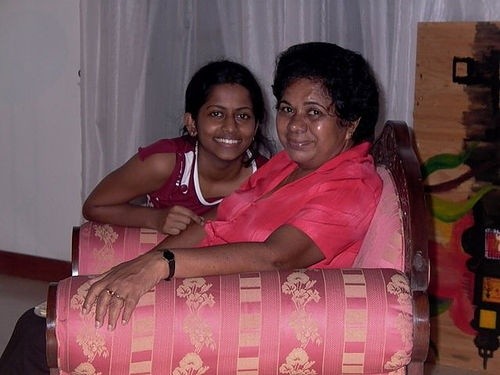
[{"xmin": 155, "ymin": 249, "xmax": 176, "ymax": 281}]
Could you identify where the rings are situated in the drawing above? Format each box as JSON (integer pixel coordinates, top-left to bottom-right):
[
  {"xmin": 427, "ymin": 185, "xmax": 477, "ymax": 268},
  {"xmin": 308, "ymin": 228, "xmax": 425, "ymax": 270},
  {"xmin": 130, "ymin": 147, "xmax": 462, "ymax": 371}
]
[{"xmin": 104, "ymin": 288, "xmax": 125, "ymax": 301}]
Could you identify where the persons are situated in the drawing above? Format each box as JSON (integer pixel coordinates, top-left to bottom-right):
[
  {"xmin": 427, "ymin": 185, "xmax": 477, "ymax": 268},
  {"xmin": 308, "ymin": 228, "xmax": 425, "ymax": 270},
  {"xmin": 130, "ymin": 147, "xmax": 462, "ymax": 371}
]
[
  {"xmin": 81, "ymin": 59, "xmax": 277, "ymax": 235},
  {"xmin": 0, "ymin": 41, "xmax": 383, "ymax": 375}
]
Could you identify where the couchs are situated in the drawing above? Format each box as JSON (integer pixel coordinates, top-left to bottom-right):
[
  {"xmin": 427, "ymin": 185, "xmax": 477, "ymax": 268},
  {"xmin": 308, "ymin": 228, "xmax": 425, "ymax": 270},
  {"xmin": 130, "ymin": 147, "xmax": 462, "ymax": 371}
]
[{"xmin": 46, "ymin": 120, "xmax": 431, "ymax": 375}]
